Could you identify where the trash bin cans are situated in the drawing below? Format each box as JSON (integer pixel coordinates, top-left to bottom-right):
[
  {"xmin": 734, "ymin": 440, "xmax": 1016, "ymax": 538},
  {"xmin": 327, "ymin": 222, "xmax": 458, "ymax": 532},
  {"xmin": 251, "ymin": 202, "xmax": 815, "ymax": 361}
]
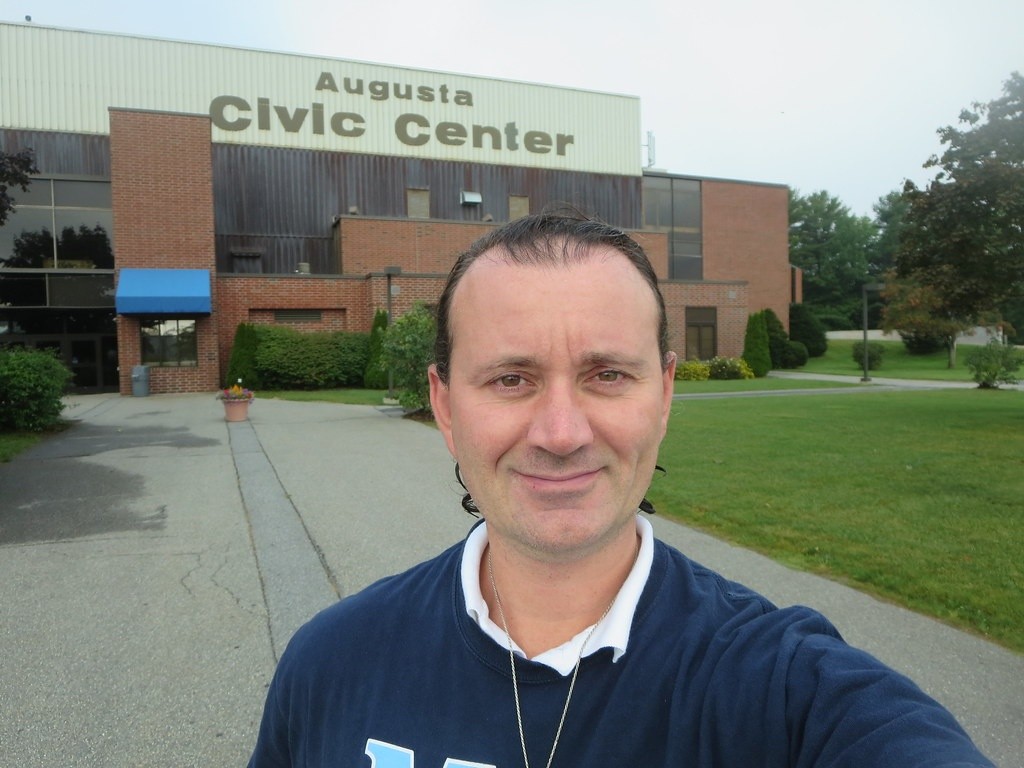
[{"xmin": 131, "ymin": 365, "xmax": 150, "ymax": 397}]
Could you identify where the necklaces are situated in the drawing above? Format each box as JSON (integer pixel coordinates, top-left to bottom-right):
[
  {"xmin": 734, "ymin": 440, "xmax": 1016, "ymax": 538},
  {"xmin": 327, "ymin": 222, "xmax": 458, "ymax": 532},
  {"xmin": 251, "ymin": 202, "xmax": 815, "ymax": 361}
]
[{"xmin": 488, "ymin": 554, "xmax": 617, "ymax": 768}]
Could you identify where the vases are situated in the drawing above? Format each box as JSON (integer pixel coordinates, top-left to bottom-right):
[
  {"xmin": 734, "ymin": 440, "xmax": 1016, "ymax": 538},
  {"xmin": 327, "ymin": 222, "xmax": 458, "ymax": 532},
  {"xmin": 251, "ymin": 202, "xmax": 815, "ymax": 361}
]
[{"xmin": 222, "ymin": 398, "xmax": 250, "ymax": 422}]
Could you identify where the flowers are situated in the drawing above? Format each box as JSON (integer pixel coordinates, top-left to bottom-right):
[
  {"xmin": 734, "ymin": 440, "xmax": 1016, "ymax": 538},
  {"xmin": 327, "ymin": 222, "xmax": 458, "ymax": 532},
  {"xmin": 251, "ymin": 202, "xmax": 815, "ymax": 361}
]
[{"xmin": 215, "ymin": 378, "xmax": 254, "ymax": 405}]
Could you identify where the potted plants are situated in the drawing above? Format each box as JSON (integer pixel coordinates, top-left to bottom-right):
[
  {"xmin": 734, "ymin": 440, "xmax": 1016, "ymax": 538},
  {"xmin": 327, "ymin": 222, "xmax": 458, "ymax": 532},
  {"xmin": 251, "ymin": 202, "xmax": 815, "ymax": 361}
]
[{"xmin": 377, "ymin": 329, "xmax": 399, "ymax": 405}]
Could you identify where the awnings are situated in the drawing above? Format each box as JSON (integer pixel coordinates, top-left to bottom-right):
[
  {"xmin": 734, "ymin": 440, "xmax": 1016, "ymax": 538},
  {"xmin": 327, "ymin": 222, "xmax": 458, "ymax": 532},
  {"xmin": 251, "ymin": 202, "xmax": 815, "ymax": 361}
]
[{"xmin": 115, "ymin": 268, "xmax": 212, "ymax": 316}]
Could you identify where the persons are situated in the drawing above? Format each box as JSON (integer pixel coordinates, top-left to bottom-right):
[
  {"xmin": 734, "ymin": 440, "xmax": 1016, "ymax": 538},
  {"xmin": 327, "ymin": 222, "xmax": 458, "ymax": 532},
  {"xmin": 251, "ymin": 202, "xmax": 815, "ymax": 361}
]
[{"xmin": 250, "ymin": 201, "xmax": 996, "ymax": 768}]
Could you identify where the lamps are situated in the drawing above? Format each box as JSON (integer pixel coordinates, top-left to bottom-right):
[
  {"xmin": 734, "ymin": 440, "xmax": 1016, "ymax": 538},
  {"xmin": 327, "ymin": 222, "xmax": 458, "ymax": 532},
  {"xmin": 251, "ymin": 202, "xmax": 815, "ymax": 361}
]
[{"xmin": 349, "ymin": 207, "xmax": 357, "ymax": 215}]
